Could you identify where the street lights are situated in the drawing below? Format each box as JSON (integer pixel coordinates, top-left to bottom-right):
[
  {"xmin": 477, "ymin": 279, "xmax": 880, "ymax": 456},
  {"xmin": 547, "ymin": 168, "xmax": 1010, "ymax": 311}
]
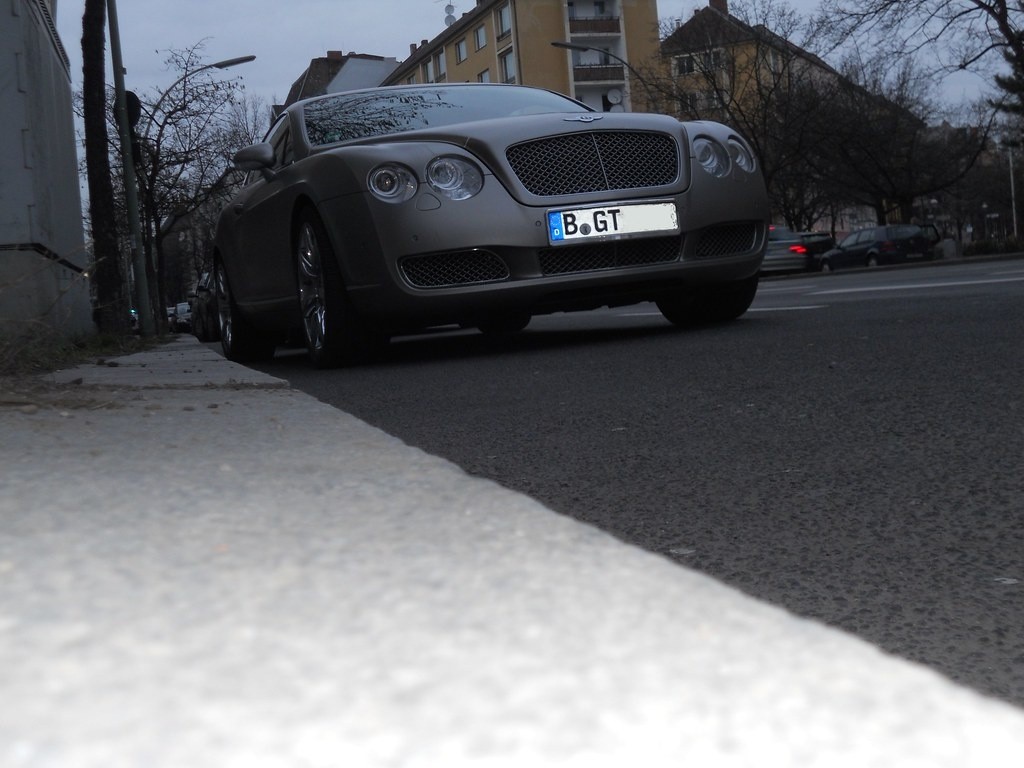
[
  {"xmin": 549, "ymin": 40, "xmax": 658, "ymax": 115},
  {"xmin": 141, "ymin": 53, "xmax": 257, "ymax": 162},
  {"xmin": 1002, "ymin": 130, "xmax": 1019, "ymax": 238}
]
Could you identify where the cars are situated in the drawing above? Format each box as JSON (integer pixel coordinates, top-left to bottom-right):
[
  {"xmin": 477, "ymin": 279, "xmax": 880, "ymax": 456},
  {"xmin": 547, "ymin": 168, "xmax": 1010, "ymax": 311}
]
[
  {"xmin": 819, "ymin": 224, "xmax": 930, "ymax": 274},
  {"xmin": 760, "ymin": 224, "xmax": 835, "ymax": 278},
  {"xmin": 131, "ymin": 272, "xmax": 220, "ymax": 343}
]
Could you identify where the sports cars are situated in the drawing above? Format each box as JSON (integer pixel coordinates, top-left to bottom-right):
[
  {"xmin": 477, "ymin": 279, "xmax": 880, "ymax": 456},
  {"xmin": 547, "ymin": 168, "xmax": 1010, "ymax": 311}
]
[{"xmin": 209, "ymin": 83, "xmax": 771, "ymax": 368}]
